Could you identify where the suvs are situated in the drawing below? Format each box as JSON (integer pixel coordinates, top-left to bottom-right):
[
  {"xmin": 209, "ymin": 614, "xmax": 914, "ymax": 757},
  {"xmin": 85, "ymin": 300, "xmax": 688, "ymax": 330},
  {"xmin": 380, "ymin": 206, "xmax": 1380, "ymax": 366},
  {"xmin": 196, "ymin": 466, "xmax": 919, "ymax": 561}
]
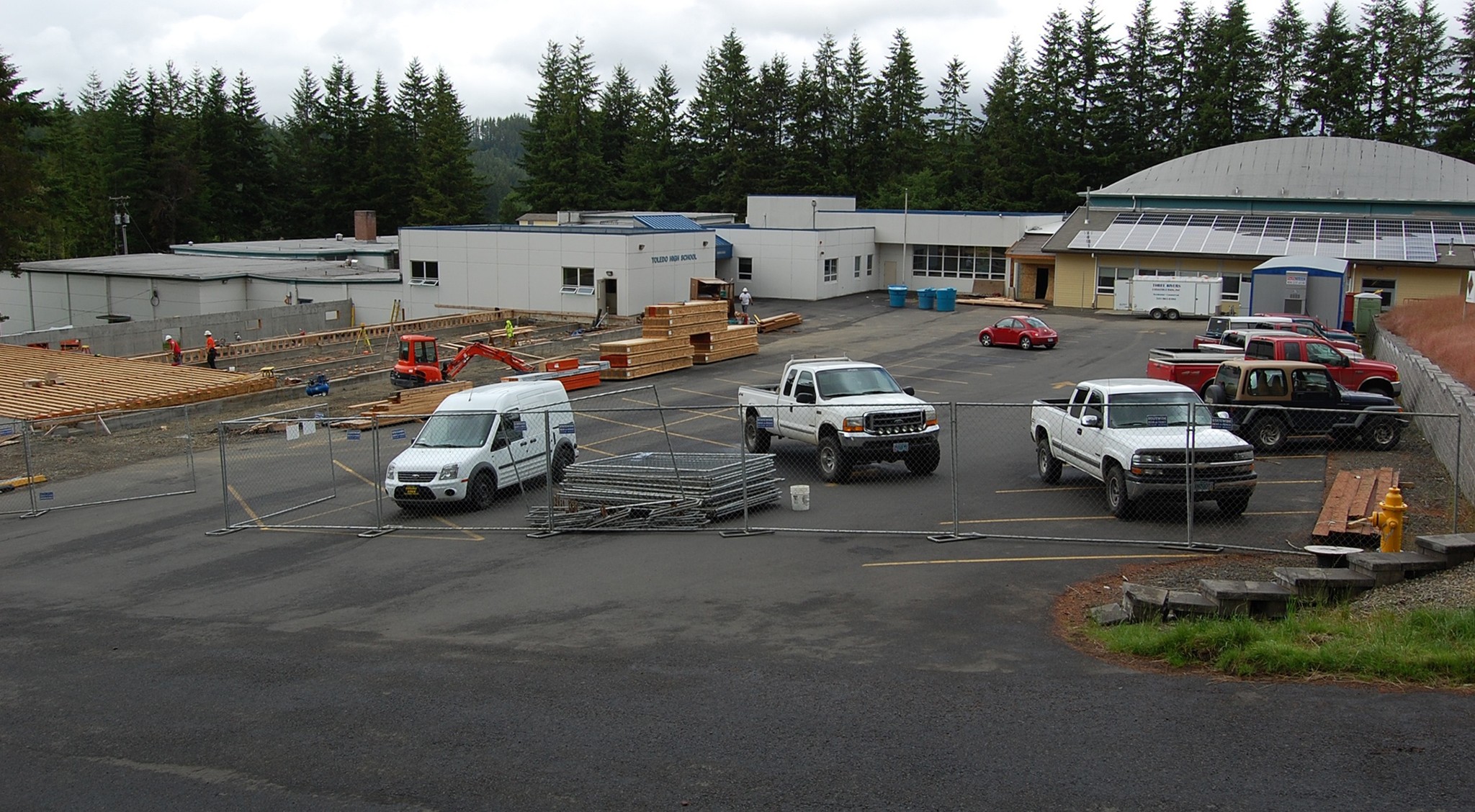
[
  {"xmin": 1205, "ymin": 316, "xmax": 1358, "ymax": 344},
  {"xmin": 1204, "ymin": 360, "xmax": 1410, "ymax": 454}
]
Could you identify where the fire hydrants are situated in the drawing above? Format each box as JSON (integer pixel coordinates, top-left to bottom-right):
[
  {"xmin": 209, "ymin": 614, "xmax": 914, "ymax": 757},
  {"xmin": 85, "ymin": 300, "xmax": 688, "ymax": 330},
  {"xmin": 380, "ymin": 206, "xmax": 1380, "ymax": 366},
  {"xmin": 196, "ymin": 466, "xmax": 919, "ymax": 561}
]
[{"xmin": 1366, "ymin": 485, "xmax": 1409, "ymax": 553}]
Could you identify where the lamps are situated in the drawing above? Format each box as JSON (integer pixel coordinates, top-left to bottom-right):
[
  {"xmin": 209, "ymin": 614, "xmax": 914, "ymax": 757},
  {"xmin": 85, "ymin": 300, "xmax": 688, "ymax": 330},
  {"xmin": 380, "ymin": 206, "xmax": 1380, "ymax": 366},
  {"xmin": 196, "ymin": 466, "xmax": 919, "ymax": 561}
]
[
  {"xmin": 607, "ymin": 271, "xmax": 613, "ymax": 276},
  {"xmin": 703, "ymin": 241, "xmax": 708, "ymax": 246},
  {"xmin": 639, "ymin": 245, "xmax": 644, "ymax": 250}
]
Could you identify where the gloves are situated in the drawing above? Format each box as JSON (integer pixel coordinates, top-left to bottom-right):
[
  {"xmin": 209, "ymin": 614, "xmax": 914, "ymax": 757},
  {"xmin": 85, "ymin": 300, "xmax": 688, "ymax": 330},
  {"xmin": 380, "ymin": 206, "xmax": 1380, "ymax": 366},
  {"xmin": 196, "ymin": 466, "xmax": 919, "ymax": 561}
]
[
  {"xmin": 741, "ymin": 299, "xmax": 742, "ymax": 301},
  {"xmin": 752, "ymin": 303, "xmax": 753, "ymax": 305}
]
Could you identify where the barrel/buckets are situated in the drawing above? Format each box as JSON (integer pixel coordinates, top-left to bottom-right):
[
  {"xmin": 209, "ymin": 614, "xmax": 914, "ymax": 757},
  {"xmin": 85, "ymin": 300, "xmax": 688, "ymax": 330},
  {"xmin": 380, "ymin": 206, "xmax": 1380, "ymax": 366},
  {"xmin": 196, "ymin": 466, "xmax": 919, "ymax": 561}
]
[
  {"xmin": 790, "ymin": 485, "xmax": 810, "ymax": 511},
  {"xmin": 917, "ymin": 288, "xmax": 936, "ymax": 309},
  {"xmin": 229, "ymin": 366, "xmax": 235, "ymax": 371},
  {"xmin": 936, "ymin": 288, "xmax": 957, "ymax": 312},
  {"xmin": 888, "ymin": 285, "xmax": 908, "ymax": 307}
]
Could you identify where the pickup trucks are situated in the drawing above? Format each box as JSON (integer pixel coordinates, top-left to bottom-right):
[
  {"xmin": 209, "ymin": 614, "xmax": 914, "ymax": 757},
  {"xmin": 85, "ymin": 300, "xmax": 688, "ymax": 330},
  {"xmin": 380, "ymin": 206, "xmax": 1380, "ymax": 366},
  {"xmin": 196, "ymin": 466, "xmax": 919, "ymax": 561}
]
[
  {"xmin": 737, "ymin": 351, "xmax": 941, "ymax": 484},
  {"xmin": 1146, "ymin": 323, "xmax": 1404, "ymax": 417},
  {"xmin": 1254, "ymin": 312, "xmax": 1350, "ymax": 334},
  {"xmin": 1029, "ymin": 378, "xmax": 1257, "ymax": 520}
]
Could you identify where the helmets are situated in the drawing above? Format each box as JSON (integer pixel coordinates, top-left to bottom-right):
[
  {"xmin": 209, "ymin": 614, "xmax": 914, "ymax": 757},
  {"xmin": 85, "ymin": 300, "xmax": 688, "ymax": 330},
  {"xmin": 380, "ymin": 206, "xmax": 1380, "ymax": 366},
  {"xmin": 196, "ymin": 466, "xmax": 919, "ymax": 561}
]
[
  {"xmin": 165, "ymin": 335, "xmax": 172, "ymax": 341},
  {"xmin": 204, "ymin": 330, "xmax": 211, "ymax": 336},
  {"xmin": 743, "ymin": 287, "xmax": 748, "ymax": 292}
]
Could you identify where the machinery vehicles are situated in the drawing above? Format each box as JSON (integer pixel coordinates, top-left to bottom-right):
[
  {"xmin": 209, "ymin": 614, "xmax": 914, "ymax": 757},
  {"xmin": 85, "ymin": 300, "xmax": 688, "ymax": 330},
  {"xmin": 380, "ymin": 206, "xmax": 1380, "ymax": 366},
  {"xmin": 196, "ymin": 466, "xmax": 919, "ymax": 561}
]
[{"xmin": 389, "ymin": 334, "xmax": 537, "ymax": 388}]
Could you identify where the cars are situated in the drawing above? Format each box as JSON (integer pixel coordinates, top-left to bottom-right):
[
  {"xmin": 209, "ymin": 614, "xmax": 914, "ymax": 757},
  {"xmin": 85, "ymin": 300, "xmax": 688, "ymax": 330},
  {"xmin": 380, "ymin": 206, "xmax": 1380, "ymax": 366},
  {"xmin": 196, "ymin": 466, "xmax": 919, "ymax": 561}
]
[{"xmin": 977, "ymin": 315, "xmax": 1059, "ymax": 350}]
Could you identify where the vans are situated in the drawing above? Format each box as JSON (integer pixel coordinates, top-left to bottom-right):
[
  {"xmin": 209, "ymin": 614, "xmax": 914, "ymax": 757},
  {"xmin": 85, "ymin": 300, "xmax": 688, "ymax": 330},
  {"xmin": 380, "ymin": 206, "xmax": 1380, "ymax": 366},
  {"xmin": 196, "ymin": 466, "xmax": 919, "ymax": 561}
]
[{"xmin": 383, "ymin": 379, "xmax": 582, "ymax": 513}]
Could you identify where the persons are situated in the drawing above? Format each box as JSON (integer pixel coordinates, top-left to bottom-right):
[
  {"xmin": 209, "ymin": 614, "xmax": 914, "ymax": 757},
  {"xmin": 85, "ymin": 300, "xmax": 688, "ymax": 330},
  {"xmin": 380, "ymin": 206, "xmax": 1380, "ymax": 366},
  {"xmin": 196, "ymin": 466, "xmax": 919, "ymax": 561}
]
[
  {"xmin": 165, "ymin": 335, "xmax": 181, "ymax": 364},
  {"xmin": 204, "ymin": 330, "xmax": 217, "ymax": 369},
  {"xmin": 739, "ymin": 287, "xmax": 753, "ymax": 313}
]
